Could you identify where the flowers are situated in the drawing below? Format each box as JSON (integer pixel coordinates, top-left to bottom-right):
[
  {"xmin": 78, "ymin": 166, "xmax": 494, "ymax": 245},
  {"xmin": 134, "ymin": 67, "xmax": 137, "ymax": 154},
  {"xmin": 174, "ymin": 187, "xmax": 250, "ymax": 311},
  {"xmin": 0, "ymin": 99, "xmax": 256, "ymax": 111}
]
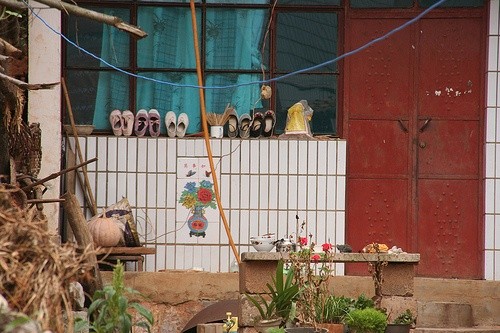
[{"xmin": 272, "ymin": 214, "xmax": 336, "ymax": 333}]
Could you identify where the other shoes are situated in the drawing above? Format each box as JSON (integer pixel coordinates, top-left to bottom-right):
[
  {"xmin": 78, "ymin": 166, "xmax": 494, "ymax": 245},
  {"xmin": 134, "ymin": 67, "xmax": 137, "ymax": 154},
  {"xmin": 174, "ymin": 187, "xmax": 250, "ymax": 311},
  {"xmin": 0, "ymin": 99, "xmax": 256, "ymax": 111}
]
[
  {"xmin": 148, "ymin": 108, "xmax": 160, "ymax": 137},
  {"xmin": 239, "ymin": 114, "xmax": 251, "ymax": 139},
  {"xmin": 121, "ymin": 110, "xmax": 134, "ymax": 136},
  {"xmin": 250, "ymin": 112, "xmax": 264, "ymax": 138},
  {"xmin": 109, "ymin": 110, "xmax": 122, "ymax": 136},
  {"xmin": 165, "ymin": 111, "xmax": 177, "ymax": 137},
  {"xmin": 134, "ymin": 109, "xmax": 149, "ymax": 137},
  {"xmin": 263, "ymin": 110, "xmax": 276, "ymax": 139},
  {"xmin": 227, "ymin": 108, "xmax": 239, "ymax": 138},
  {"xmin": 176, "ymin": 113, "xmax": 189, "ymax": 138}
]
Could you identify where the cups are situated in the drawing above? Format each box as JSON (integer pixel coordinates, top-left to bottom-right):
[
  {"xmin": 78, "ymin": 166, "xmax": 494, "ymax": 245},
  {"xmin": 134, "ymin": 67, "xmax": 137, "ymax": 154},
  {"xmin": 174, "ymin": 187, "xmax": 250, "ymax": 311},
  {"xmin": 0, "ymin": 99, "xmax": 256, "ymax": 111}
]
[{"xmin": 211, "ymin": 125, "xmax": 223, "ymax": 138}]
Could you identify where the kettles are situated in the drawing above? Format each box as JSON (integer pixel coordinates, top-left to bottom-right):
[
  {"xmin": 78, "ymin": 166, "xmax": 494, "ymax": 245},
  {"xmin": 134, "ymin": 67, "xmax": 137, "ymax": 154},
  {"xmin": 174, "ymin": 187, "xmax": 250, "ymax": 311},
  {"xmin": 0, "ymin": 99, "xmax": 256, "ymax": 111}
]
[{"xmin": 273, "ymin": 237, "xmax": 296, "ymax": 252}]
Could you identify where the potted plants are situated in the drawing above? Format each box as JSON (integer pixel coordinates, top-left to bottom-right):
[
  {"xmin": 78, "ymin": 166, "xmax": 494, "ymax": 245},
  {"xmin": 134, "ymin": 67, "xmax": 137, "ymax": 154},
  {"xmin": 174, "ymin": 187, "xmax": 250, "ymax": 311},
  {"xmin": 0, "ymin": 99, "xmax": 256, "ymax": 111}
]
[
  {"xmin": 246, "ymin": 258, "xmax": 298, "ymax": 333},
  {"xmin": 313, "ymin": 296, "xmax": 419, "ymax": 333}
]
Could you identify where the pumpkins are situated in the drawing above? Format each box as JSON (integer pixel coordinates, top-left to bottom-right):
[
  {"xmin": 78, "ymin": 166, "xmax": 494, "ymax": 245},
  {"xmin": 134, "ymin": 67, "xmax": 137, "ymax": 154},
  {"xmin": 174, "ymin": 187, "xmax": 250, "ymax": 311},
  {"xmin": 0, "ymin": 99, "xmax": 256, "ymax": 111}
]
[{"xmin": 87, "ymin": 207, "xmax": 121, "ymax": 247}]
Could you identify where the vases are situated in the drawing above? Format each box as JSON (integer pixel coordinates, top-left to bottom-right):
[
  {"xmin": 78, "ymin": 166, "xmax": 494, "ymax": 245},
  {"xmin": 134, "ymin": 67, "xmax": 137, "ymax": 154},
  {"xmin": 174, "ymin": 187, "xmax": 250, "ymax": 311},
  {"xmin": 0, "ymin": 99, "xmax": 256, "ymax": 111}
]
[{"xmin": 284, "ymin": 326, "xmax": 329, "ymax": 333}]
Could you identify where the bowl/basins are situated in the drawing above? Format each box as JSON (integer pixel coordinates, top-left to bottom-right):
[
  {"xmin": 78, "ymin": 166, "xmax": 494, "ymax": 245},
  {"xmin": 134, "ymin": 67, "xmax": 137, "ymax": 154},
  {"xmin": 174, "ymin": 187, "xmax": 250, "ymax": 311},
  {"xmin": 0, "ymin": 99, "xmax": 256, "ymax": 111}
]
[{"xmin": 251, "ymin": 234, "xmax": 275, "ymax": 252}]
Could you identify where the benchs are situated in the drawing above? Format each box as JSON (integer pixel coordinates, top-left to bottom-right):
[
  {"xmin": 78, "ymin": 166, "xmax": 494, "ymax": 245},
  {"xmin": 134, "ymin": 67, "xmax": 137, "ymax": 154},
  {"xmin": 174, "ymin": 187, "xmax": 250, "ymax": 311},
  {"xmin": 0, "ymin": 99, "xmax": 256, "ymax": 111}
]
[{"xmin": 241, "ymin": 251, "xmax": 422, "ymax": 296}]
